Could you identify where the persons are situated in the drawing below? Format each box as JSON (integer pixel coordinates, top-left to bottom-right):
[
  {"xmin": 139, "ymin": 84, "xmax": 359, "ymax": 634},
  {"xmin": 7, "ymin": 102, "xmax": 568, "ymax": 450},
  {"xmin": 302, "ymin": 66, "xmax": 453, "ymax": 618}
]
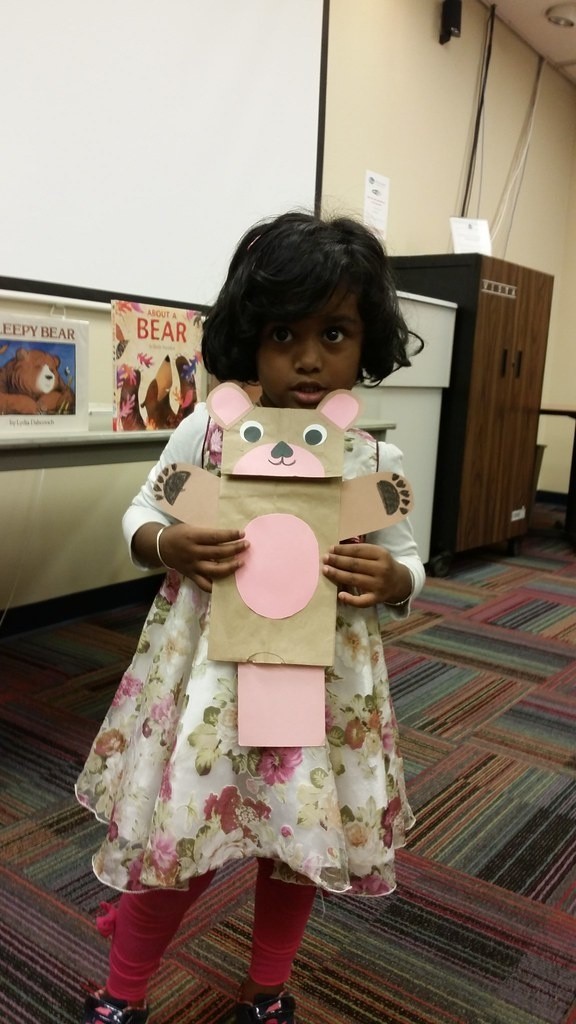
[{"xmin": 73, "ymin": 205, "xmax": 426, "ymax": 1024}]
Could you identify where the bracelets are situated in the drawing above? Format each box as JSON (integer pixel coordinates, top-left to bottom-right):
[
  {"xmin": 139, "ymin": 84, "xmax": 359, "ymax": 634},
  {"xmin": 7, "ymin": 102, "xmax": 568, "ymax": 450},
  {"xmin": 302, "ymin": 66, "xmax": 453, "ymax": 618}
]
[
  {"xmin": 157, "ymin": 526, "xmax": 175, "ymax": 571},
  {"xmin": 384, "ymin": 570, "xmax": 414, "ymax": 606}
]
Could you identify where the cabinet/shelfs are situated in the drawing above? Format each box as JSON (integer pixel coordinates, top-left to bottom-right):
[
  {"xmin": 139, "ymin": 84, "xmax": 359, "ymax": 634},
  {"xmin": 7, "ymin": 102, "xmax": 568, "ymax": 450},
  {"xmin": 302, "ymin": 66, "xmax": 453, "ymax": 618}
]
[{"xmin": 388, "ymin": 252, "xmax": 554, "ymax": 578}]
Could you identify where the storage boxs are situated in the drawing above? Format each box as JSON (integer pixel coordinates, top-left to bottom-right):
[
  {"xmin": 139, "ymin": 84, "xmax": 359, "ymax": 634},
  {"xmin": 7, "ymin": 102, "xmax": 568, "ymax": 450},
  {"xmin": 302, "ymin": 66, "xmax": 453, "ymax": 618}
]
[{"xmin": 362, "ymin": 290, "xmax": 458, "ymax": 388}]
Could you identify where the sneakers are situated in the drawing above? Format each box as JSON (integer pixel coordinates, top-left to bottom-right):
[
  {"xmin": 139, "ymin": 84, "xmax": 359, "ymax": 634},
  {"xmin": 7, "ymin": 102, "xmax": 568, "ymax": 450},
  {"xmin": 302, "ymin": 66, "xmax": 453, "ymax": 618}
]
[
  {"xmin": 82, "ymin": 988, "xmax": 150, "ymax": 1024},
  {"xmin": 234, "ymin": 979, "xmax": 297, "ymax": 1024}
]
